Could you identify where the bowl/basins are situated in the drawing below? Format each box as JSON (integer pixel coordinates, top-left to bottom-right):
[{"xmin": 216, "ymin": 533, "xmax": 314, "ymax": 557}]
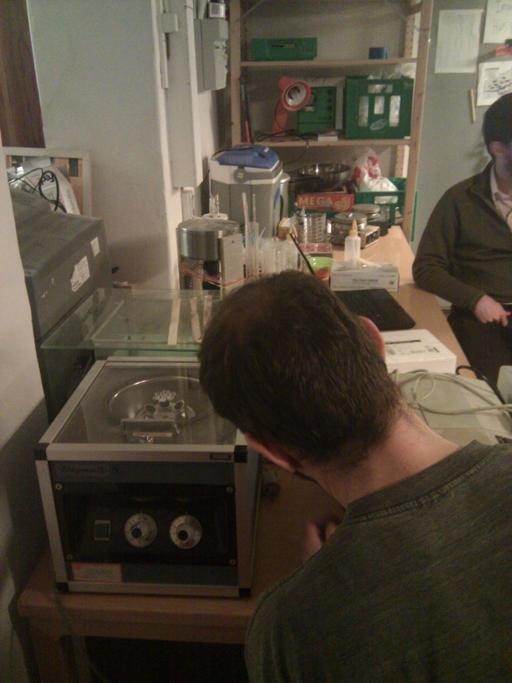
[{"xmin": 297, "ymin": 162, "xmax": 351, "ymax": 191}]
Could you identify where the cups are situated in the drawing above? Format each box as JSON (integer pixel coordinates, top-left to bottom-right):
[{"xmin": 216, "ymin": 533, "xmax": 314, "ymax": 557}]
[{"xmin": 303, "ymin": 212, "xmax": 327, "ymax": 251}]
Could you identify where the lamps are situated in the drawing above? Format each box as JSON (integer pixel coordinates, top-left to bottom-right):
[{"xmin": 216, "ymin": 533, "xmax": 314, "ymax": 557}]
[{"xmin": 268, "ymin": 74, "xmax": 312, "ymax": 139}]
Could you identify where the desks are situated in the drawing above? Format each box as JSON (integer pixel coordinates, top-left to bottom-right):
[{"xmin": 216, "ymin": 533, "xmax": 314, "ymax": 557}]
[{"xmin": 16, "ymin": 225, "xmax": 511, "ymax": 683}]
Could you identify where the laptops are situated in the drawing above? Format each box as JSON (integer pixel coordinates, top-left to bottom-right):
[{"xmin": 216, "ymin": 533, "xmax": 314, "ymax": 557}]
[{"xmin": 290, "ymin": 233, "xmax": 416, "ymax": 332}]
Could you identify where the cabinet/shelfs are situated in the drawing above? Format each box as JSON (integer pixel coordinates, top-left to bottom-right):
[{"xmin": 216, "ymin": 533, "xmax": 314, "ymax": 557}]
[{"xmin": 230, "ymin": 1, "xmax": 433, "ymax": 243}]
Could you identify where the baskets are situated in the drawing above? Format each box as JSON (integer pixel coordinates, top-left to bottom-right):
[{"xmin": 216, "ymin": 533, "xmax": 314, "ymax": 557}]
[
  {"xmin": 297, "ymin": 86, "xmax": 337, "ymax": 134},
  {"xmin": 343, "ymin": 75, "xmax": 414, "ymax": 140},
  {"xmin": 351, "ymin": 176, "xmax": 418, "ymax": 242},
  {"xmin": 251, "ymin": 37, "xmax": 317, "ymax": 60}
]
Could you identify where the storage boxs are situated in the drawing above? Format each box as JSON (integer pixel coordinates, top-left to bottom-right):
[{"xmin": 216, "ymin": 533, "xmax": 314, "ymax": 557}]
[
  {"xmin": 17, "ymin": 209, "xmax": 114, "ymax": 340},
  {"xmin": 38, "ymin": 287, "xmax": 114, "ymax": 424}
]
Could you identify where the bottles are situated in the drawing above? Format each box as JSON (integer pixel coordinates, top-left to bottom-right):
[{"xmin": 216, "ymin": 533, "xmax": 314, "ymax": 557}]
[{"xmin": 343, "ymin": 219, "xmax": 362, "ymax": 268}]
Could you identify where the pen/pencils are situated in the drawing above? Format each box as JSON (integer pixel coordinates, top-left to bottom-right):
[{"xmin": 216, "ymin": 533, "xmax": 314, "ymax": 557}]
[{"xmin": 469, "ymin": 89, "xmax": 476, "ymax": 123}]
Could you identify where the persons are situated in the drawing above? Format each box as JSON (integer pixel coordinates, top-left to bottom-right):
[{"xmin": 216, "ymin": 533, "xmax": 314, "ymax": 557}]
[
  {"xmin": 411, "ymin": 92, "xmax": 511, "ymax": 388},
  {"xmin": 199, "ymin": 269, "xmax": 511, "ymax": 683}
]
